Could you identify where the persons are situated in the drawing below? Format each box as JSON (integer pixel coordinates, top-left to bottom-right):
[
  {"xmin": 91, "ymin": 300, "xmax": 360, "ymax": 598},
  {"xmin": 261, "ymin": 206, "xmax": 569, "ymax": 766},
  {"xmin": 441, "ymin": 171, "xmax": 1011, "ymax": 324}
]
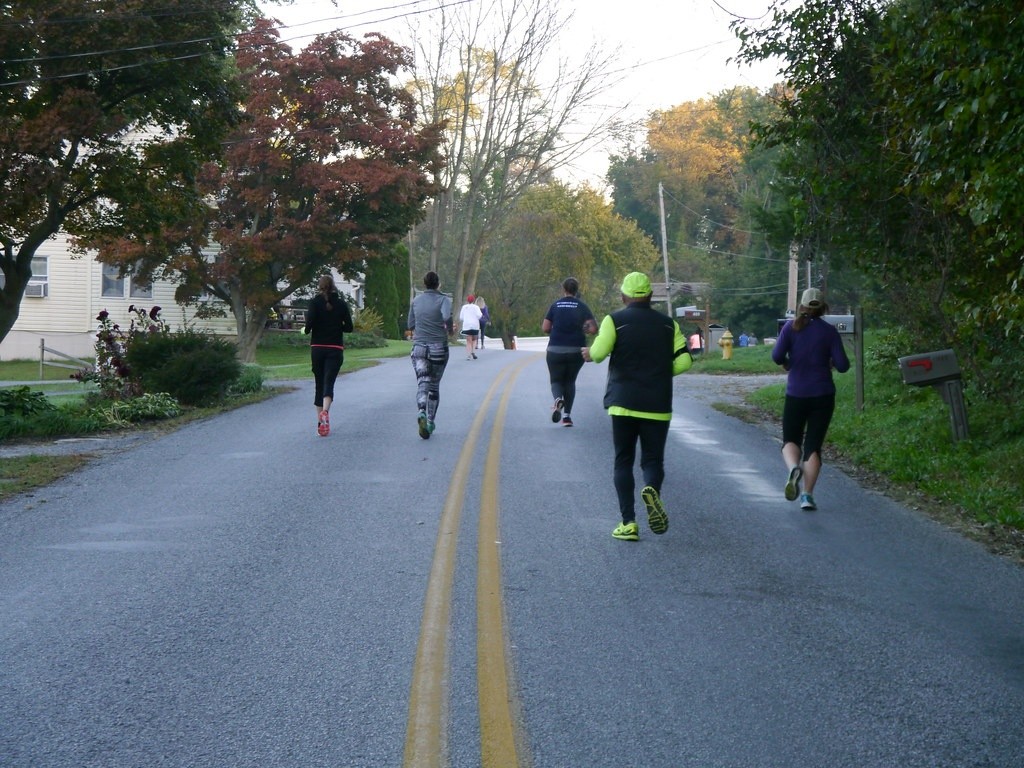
[
  {"xmin": 474, "ymin": 297, "xmax": 491, "ymax": 349},
  {"xmin": 407, "ymin": 271, "xmax": 454, "ymax": 439},
  {"xmin": 459, "ymin": 295, "xmax": 483, "ymax": 361},
  {"xmin": 739, "ymin": 331, "xmax": 749, "ymax": 347},
  {"xmin": 301, "ymin": 274, "xmax": 354, "ymax": 436},
  {"xmin": 543, "ymin": 278, "xmax": 597, "ymax": 426},
  {"xmin": 581, "ymin": 273, "xmax": 693, "ymax": 540},
  {"xmin": 771, "ymin": 287, "xmax": 851, "ymax": 510},
  {"xmin": 748, "ymin": 333, "xmax": 758, "ymax": 346}
]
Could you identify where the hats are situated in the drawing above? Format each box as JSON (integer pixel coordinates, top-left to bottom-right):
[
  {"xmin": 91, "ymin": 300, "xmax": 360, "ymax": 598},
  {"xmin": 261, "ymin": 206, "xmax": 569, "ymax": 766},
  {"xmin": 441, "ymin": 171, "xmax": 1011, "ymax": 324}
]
[
  {"xmin": 621, "ymin": 272, "xmax": 651, "ymax": 298},
  {"xmin": 801, "ymin": 287, "xmax": 825, "ymax": 309},
  {"xmin": 468, "ymin": 295, "xmax": 474, "ymax": 302}
]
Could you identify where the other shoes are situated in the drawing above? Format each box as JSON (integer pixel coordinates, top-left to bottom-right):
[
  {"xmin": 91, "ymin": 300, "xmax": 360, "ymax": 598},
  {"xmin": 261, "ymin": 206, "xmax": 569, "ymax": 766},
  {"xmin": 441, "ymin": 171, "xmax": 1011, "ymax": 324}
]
[
  {"xmin": 471, "ymin": 353, "xmax": 477, "ymax": 359},
  {"xmin": 467, "ymin": 356, "xmax": 470, "ymax": 360}
]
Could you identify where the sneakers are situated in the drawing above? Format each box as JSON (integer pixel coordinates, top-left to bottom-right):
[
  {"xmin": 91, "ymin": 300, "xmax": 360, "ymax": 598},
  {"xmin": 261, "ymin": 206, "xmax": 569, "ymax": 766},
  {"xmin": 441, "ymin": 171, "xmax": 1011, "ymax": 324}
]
[
  {"xmin": 318, "ymin": 411, "xmax": 330, "ymax": 436},
  {"xmin": 800, "ymin": 493, "xmax": 816, "ymax": 509},
  {"xmin": 563, "ymin": 416, "xmax": 573, "ymax": 426},
  {"xmin": 642, "ymin": 486, "xmax": 669, "ymax": 534},
  {"xmin": 552, "ymin": 397, "xmax": 565, "ymax": 422},
  {"xmin": 785, "ymin": 467, "xmax": 803, "ymax": 501},
  {"xmin": 417, "ymin": 410, "xmax": 429, "ymax": 439},
  {"xmin": 612, "ymin": 522, "xmax": 639, "ymax": 540},
  {"xmin": 427, "ymin": 423, "xmax": 435, "ymax": 433}
]
